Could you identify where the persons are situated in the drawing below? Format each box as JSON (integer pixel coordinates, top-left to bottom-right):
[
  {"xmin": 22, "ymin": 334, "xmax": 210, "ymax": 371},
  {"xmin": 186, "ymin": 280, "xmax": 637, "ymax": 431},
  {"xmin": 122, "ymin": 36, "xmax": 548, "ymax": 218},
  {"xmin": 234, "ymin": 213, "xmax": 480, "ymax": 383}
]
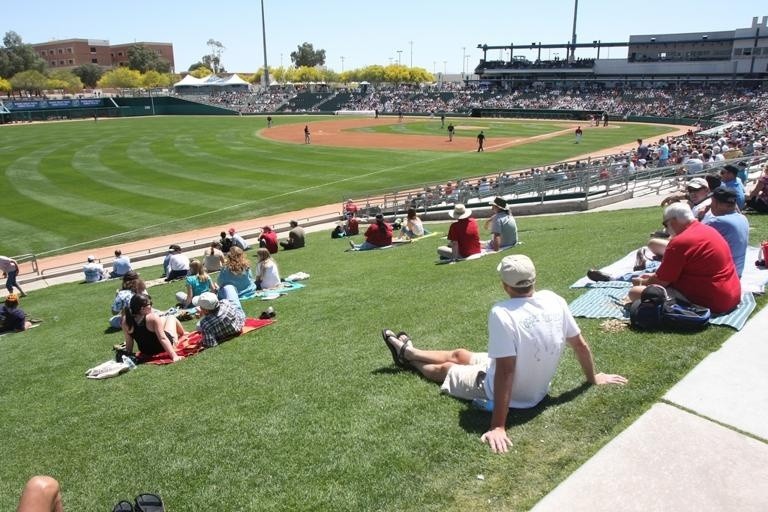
[
  {"xmin": 305, "ymin": 125, "xmax": 312, "ymax": 144},
  {"xmin": 349, "ymin": 214, "xmax": 394, "ymax": 250},
  {"xmin": 0, "ymin": 292, "xmax": 32, "ymax": 332},
  {"xmin": 437, "ymin": 203, "xmax": 481, "ymax": 264},
  {"xmin": 647, "ymin": 186, "xmax": 750, "ymax": 280},
  {"xmin": 85, "ymin": 220, "xmax": 308, "ymax": 362},
  {"xmin": 483, "ymin": 197, "xmax": 518, "ymax": 252},
  {"xmin": 209, "ymin": 83, "xmax": 768, "ymax": 118},
  {"xmin": 17, "ymin": 474, "xmax": 63, "ymax": 512},
  {"xmin": 267, "ymin": 116, "xmax": 272, "ymax": 128},
  {"xmin": 628, "ymin": 200, "xmax": 741, "ymax": 316},
  {"xmin": 396, "ymin": 206, "xmax": 424, "ymax": 239},
  {"xmin": 341, "ymin": 110, "xmax": 767, "ymax": 211},
  {"xmin": 344, "ymin": 213, "xmax": 359, "ymax": 235},
  {"xmin": 0, "ymin": 255, "xmax": 28, "ymax": 297},
  {"xmin": 380, "ymin": 253, "xmax": 629, "ymax": 455}
]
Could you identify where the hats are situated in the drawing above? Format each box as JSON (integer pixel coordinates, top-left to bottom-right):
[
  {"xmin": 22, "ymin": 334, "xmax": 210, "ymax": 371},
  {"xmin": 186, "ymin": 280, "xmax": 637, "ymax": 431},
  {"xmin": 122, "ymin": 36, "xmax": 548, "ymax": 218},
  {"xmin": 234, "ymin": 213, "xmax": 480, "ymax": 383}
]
[
  {"xmin": 192, "ymin": 292, "xmax": 219, "ymax": 310},
  {"xmin": 448, "ymin": 204, "xmax": 473, "ymax": 219},
  {"xmin": 489, "ymin": 197, "xmax": 510, "ymax": 211},
  {"xmin": 685, "ymin": 177, "xmax": 709, "ymax": 190},
  {"xmin": 707, "ymin": 187, "xmax": 737, "ymax": 203},
  {"xmin": 228, "ymin": 228, "xmax": 235, "ymax": 233},
  {"xmin": 6, "ymin": 293, "xmax": 19, "ymax": 304},
  {"xmin": 639, "ymin": 284, "xmax": 668, "ymax": 309},
  {"xmin": 123, "ymin": 271, "xmax": 138, "ymax": 281},
  {"xmin": 497, "ymin": 254, "xmax": 536, "ymax": 288}
]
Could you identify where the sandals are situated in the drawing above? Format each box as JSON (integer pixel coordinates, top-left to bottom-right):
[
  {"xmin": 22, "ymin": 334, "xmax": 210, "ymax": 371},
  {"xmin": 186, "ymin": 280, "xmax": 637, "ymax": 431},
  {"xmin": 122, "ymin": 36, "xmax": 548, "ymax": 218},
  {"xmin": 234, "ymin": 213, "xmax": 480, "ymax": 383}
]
[{"xmin": 382, "ymin": 329, "xmax": 411, "ymax": 366}]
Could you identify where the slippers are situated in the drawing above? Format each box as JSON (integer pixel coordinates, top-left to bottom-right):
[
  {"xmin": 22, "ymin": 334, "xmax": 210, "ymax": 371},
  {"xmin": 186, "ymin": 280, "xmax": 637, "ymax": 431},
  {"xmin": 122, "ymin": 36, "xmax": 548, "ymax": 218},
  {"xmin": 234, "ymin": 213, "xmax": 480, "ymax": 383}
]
[{"xmin": 112, "ymin": 494, "xmax": 164, "ymax": 512}]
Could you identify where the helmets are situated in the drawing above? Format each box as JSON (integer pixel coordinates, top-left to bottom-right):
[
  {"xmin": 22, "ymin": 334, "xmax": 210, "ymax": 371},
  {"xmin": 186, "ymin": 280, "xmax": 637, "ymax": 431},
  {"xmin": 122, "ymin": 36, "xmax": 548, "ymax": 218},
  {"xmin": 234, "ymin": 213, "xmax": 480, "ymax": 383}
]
[{"xmin": 88, "ymin": 256, "xmax": 94, "ymax": 261}]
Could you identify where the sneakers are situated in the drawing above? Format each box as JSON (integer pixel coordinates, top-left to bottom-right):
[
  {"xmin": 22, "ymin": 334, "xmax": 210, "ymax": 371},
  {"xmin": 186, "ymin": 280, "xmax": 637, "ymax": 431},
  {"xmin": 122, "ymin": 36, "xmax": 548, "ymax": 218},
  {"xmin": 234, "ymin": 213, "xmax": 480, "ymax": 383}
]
[
  {"xmin": 260, "ymin": 312, "xmax": 275, "ymax": 319},
  {"xmin": 588, "ymin": 269, "xmax": 610, "ymax": 281},
  {"xmin": 113, "ymin": 341, "xmax": 127, "ymax": 351},
  {"xmin": 634, "ymin": 248, "xmax": 648, "ymax": 271}
]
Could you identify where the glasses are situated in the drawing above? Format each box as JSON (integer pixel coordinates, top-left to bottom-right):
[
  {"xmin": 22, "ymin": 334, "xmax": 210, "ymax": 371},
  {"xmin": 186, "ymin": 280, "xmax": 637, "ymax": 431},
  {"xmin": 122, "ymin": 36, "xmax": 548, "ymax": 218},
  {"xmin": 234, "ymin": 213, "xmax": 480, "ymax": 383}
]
[{"xmin": 143, "ymin": 302, "xmax": 152, "ymax": 307}]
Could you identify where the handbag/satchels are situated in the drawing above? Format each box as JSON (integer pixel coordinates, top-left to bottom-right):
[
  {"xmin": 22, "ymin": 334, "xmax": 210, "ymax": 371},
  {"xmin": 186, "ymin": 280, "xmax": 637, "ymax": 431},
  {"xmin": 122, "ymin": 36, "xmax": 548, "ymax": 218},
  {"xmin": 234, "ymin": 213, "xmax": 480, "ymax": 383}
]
[{"xmin": 630, "ymin": 299, "xmax": 710, "ymax": 333}]
